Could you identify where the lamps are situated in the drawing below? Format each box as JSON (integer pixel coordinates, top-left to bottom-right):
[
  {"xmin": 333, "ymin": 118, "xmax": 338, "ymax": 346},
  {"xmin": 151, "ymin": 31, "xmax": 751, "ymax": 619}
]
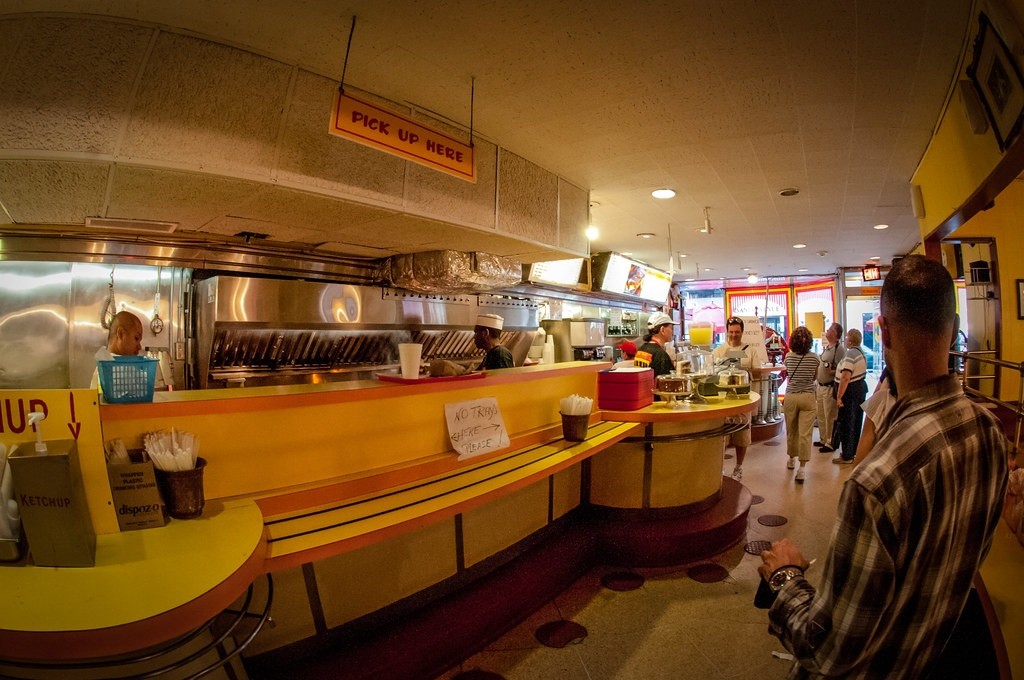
[
  {"xmin": 957, "ymin": 80, "xmax": 986, "ymax": 134},
  {"xmin": 909, "ymin": 185, "xmax": 924, "ymax": 219}
]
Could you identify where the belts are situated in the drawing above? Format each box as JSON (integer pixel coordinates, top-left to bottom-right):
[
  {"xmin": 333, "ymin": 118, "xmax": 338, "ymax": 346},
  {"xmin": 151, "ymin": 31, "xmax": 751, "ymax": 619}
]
[{"xmin": 819, "ymin": 381, "xmax": 834, "ymax": 386}]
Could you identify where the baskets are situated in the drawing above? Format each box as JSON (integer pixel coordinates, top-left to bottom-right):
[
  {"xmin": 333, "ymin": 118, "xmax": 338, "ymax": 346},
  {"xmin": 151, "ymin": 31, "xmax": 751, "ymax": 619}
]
[{"xmin": 96, "ymin": 355, "xmax": 160, "ymax": 403}]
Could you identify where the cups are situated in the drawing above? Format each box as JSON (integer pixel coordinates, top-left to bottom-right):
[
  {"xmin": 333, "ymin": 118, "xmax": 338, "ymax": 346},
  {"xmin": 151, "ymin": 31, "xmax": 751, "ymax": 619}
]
[
  {"xmin": 558, "ymin": 412, "xmax": 590, "ymax": 441},
  {"xmin": 541, "ymin": 334, "xmax": 555, "ymax": 364},
  {"xmin": 398, "ymin": 343, "xmax": 423, "ymax": 379},
  {"xmin": 154, "ymin": 456, "xmax": 209, "ymax": 520}
]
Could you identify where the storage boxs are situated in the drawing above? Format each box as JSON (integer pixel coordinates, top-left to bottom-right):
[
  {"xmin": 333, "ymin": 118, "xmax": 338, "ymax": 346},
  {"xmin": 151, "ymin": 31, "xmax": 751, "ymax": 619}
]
[
  {"xmin": 106, "ymin": 448, "xmax": 164, "ymax": 532},
  {"xmin": 596, "ymin": 369, "xmax": 654, "ymax": 411}
]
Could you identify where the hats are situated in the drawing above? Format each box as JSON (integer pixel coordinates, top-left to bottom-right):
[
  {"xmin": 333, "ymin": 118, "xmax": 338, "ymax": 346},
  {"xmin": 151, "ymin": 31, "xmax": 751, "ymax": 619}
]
[
  {"xmin": 476, "ymin": 314, "xmax": 504, "ymax": 330},
  {"xmin": 647, "ymin": 312, "xmax": 681, "ymax": 329}
]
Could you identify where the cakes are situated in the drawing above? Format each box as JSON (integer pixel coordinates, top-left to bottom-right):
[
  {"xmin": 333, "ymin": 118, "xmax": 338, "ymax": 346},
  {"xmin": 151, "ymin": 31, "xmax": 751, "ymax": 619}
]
[
  {"xmin": 655, "ymin": 378, "xmax": 689, "ymax": 393},
  {"xmin": 718, "ymin": 373, "xmax": 750, "ymax": 385},
  {"xmin": 676, "ymin": 360, "xmax": 692, "ymax": 373}
]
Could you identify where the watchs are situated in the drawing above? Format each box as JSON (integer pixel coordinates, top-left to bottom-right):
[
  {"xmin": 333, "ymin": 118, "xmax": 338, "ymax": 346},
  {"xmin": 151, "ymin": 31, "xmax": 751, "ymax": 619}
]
[{"xmin": 769, "ymin": 569, "xmax": 803, "ymax": 597}]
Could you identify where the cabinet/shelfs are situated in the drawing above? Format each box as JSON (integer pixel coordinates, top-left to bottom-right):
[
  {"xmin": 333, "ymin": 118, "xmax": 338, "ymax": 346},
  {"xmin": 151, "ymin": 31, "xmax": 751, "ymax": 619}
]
[{"xmin": 666, "ymin": 207, "xmax": 711, "ymax": 278}]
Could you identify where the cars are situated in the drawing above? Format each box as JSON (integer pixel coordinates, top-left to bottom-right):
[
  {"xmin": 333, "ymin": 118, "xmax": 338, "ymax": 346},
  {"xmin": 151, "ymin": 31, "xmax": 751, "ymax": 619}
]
[
  {"xmin": 959, "ymin": 330, "xmax": 968, "ymax": 350},
  {"xmin": 861, "ymin": 346, "xmax": 884, "ymax": 368}
]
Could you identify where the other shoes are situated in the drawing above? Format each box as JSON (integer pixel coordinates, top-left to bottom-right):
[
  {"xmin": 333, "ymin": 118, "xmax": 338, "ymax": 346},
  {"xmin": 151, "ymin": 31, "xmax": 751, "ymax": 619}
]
[
  {"xmin": 832, "ymin": 458, "xmax": 854, "ymax": 464},
  {"xmin": 795, "ymin": 470, "xmax": 805, "ymax": 480},
  {"xmin": 787, "ymin": 459, "xmax": 795, "ymax": 469},
  {"xmin": 814, "ymin": 442, "xmax": 825, "ymax": 446},
  {"xmin": 732, "ymin": 468, "xmax": 743, "ymax": 481},
  {"xmin": 819, "ymin": 446, "xmax": 834, "ymax": 453}
]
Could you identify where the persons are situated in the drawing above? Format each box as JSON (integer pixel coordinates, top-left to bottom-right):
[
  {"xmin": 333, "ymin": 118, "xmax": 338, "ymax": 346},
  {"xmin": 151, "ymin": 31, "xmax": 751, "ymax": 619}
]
[
  {"xmin": 709, "ymin": 316, "xmax": 762, "ymax": 481},
  {"xmin": 780, "ymin": 322, "xmax": 898, "ymax": 483},
  {"xmin": 611, "ymin": 310, "xmax": 676, "ymax": 401},
  {"xmin": 756, "ymin": 255, "xmax": 1013, "ymax": 680},
  {"xmin": 90, "ymin": 311, "xmax": 142, "ymax": 394},
  {"xmin": 473, "ymin": 313, "xmax": 513, "ymax": 371}
]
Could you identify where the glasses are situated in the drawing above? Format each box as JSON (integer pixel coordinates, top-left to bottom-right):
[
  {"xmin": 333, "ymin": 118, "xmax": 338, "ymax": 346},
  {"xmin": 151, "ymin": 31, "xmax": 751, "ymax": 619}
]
[{"xmin": 728, "ymin": 318, "xmax": 742, "ymax": 324}]
